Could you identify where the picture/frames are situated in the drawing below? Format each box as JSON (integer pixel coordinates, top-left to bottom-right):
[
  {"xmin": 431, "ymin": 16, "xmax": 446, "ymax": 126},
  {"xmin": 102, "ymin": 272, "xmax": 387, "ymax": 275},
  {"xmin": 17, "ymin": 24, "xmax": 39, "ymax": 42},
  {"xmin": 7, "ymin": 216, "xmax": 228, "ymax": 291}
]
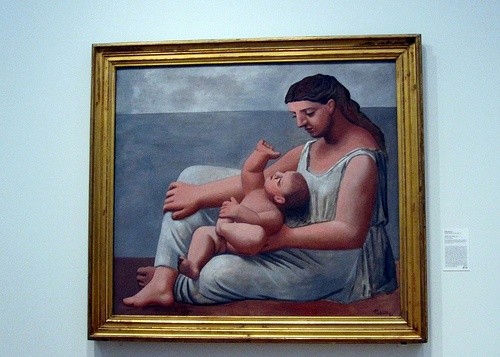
[{"xmin": 84, "ymin": 31, "xmax": 429, "ymax": 346}]
